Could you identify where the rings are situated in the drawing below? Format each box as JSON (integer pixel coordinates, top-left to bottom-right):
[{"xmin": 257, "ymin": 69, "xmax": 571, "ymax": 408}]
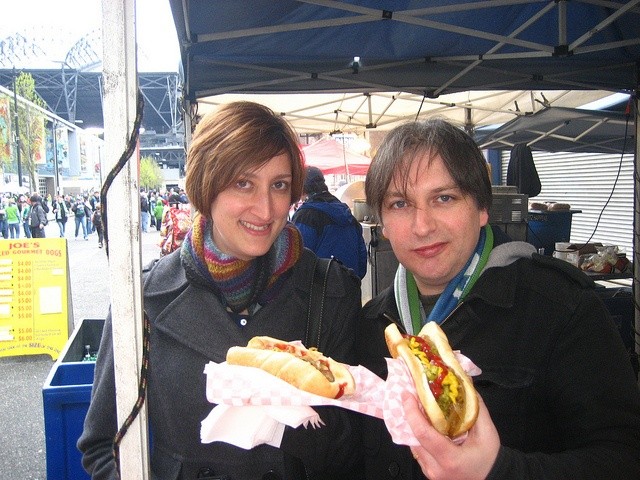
[{"xmin": 413, "ymin": 453, "xmax": 419, "ymax": 460}]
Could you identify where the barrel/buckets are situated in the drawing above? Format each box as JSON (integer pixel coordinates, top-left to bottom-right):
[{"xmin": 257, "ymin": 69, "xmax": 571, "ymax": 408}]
[
  {"xmin": 528, "ymin": 213, "xmax": 572, "ymax": 257},
  {"xmin": 353, "ymin": 198, "xmax": 372, "ymax": 222}
]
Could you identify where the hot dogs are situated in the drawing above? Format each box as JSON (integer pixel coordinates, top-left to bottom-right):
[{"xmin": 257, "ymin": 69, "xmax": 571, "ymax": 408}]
[
  {"xmin": 382, "ymin": 318, "xmax": 480, "ymax": 438},
  {"xmin": 225, "ymin": 336, "xmax": 357, "ymax": 403}
]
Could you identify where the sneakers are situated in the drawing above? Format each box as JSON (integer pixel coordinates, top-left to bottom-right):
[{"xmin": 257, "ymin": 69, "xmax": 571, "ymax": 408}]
[
  {"xmin": 84, "ymin": 236, "xmax": 88, "ymax": 240},
  {"xmin": 98, "ymin": 243, "xmax": 103, "ymax": 248}
]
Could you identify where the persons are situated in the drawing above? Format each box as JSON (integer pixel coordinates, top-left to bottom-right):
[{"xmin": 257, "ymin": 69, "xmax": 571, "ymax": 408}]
[
  {"xmin": 0, "ymin": 189, "xmax": 106, "ymax": 245},
  {"xmin": 291, "ymin": 164, "xmax": 368, "ymax": 281},
  {"xmin": 356, "ymin": 116, "xmax": 639, "ymax": 479},
  {"xmin": 139, "ymin": 179, "xmax": 189, "ymax": 235},
  {"xmin": 77, "ymin": 101, "xmax": 363, "ymax": 480}
]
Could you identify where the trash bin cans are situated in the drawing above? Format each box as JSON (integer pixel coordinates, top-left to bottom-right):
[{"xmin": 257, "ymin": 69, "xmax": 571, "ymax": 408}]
[{"xmin": 42, "ymin": 315, "xmax": 107, "ymax": 480}]
